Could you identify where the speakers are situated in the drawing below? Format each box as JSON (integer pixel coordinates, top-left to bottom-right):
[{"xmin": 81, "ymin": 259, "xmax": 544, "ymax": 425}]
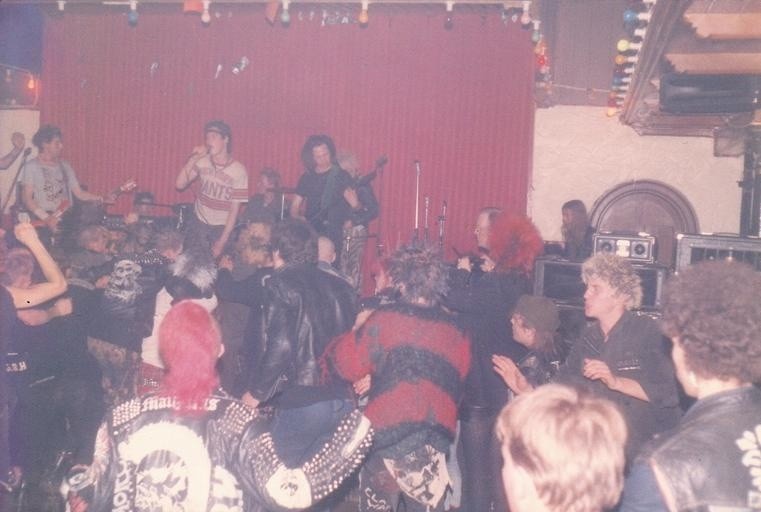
[
  {"xmin": 531, "ymin": 260, "xmax": 668, "ymax": 314},
  {"xmin": 675, "ymin": 233, "xmax": 761, "ymax": 277},
  {"xmin": 592, "ymin": 232, "xmax": 657, "ymax": 265}
]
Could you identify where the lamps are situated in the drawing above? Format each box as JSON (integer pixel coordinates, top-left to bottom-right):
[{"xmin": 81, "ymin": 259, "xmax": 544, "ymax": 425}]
[
  {"xmin": 279, "ymin": 0, "xmax": 293, "ymax": 24},
  {"xmin": 125, "ymin": 0, "xmax": 140, "ymax": 26},
  {"xmin": 357, "ymin": 0, "xmax": 370, "ymax": 23},
  {"xmin": 604, "ymin": 0, "xmax": 655, "ymax": 117},
  {"xmin": 200, "ymin": 0, "xmax": 211, "ymax": 24},
  {"xmin": 442, "ymin": 0, "xmax": 456, "ymax": 28},
  {"xmin": 513, "ymin": 1, "xmax": 555, "ymax": 98}
]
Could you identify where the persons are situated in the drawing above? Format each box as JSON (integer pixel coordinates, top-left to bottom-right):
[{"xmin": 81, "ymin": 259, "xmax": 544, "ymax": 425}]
[{"xmin": 1, "ymin": 120, "xmax": 761, "ymax": 510}]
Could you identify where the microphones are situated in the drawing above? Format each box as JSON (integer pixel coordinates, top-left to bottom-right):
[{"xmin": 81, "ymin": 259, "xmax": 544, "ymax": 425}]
[
  {"xmin": 424, "ymin": 193, "xmax": 430, "ymax": 208},
  {"xmin": 191, "ymin": 146, "xmax": 211, "ymax": 157},
  {"xmin": 414, "ymin": 160, "xmax": 422, "ymax": 176},
  {"xmin": 442, "ymin": 200, "xmax": 448, "ymax": 215}
]
